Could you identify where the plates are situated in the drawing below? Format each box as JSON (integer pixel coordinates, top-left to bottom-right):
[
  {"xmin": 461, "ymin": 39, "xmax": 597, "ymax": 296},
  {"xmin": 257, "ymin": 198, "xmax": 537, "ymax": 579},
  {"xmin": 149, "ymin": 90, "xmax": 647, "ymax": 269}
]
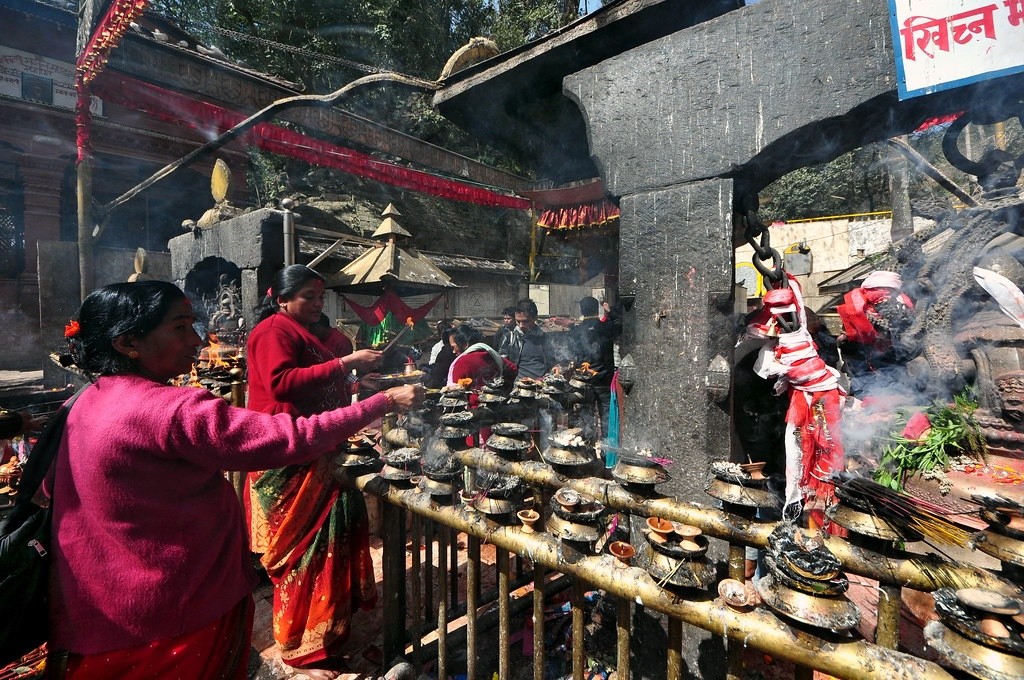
[{"xmin": 372, "ymin": 371, "xmax": 425, "ymax": 382}]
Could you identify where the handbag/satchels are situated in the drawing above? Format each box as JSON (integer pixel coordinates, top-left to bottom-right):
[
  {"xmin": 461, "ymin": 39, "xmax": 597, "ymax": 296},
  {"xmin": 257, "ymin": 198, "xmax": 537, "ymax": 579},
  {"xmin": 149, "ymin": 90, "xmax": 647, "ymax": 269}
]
[{"xmin": 0, "ymin": 373, "xmax": 97, "ymax": 670}]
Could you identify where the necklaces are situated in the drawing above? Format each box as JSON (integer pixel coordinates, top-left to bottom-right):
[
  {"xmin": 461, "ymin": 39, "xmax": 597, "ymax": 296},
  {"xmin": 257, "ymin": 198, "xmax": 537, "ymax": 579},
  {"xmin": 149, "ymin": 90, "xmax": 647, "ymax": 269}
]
[{"xmin": 310, "ymin": 330, "xmax": 336, "ymax": 359}]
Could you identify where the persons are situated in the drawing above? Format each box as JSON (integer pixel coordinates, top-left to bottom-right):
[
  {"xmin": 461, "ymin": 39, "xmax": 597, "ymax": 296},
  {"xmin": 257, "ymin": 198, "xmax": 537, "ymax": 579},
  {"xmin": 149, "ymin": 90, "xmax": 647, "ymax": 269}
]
[
  {"xmin": 244, "ymin": 266, "xmax": 384, "ymax": 680},
  {"xmin": 15, "ymin": 280, "xmax": 427, "ymax": 679},
  {"xmin": 420, "ymin": 288, "xmax": 621, "ymax": 469}
]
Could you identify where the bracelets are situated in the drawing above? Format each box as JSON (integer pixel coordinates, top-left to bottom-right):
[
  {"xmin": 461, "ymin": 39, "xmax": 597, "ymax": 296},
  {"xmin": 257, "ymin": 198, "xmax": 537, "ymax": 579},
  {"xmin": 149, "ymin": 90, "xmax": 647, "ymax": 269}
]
[
  {"xmin": 338, "ymin": 357, "xmax": 346, "ymax": 374},
  {"xmin": 382, "ymin": 390, "xmax": 395, "ymax": 409}
]
